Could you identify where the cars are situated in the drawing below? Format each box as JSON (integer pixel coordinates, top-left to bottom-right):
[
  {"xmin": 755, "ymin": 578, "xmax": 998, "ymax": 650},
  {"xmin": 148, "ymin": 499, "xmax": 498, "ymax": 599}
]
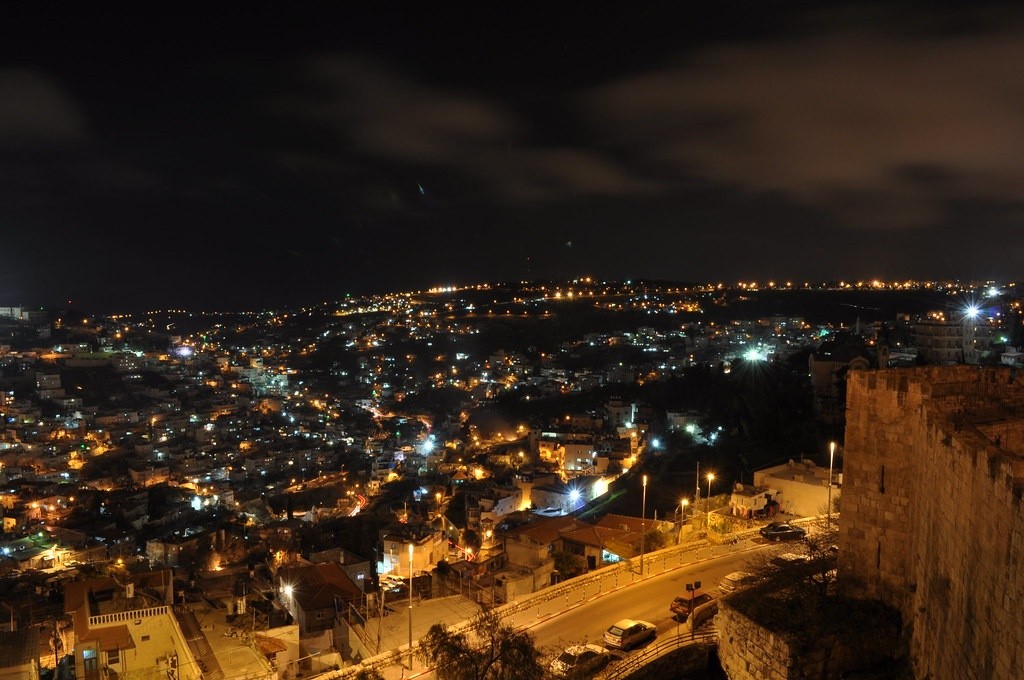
[
  {"xmin": 767, "ymin": 553, "xmax": 810, "ymax": 574},
  {"xmin": 669, "ymin": 591, "xmax": 718, "ymax": 620},
  {"xmin": 759, "ymin": 522, "xmax": 807, "ymax": 542},
  {"xmin": 719, "ymin": 571, "xmax": 765, "ymax": 594},
  {"xmin": 603, "ymin": 619, "xmax": 658, "ymax": 651},
  {"xmin": 549, "ymin": 643, "xmax": 610, "ymax": 679}
]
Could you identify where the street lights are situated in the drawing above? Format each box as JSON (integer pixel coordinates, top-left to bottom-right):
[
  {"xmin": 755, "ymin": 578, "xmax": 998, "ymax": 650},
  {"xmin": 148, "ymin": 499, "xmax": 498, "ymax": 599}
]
[
  {"xmin": 707, "ymin": 472, "xmax": 715, "ymax": 512},
  {"xmin": 828, "ymin": 442, "xmax": 835, "ymax": 527},
  {"xmin": 408, "ymin": 544, "xmax": 413, "ymax": 671},
  {"xmin": 639, "ymin": 476, "xmax": 648, "ymax": 575},
  {"xmin": 674, "ymin": 498, "xmax": 688, "ymax": 524}
]
